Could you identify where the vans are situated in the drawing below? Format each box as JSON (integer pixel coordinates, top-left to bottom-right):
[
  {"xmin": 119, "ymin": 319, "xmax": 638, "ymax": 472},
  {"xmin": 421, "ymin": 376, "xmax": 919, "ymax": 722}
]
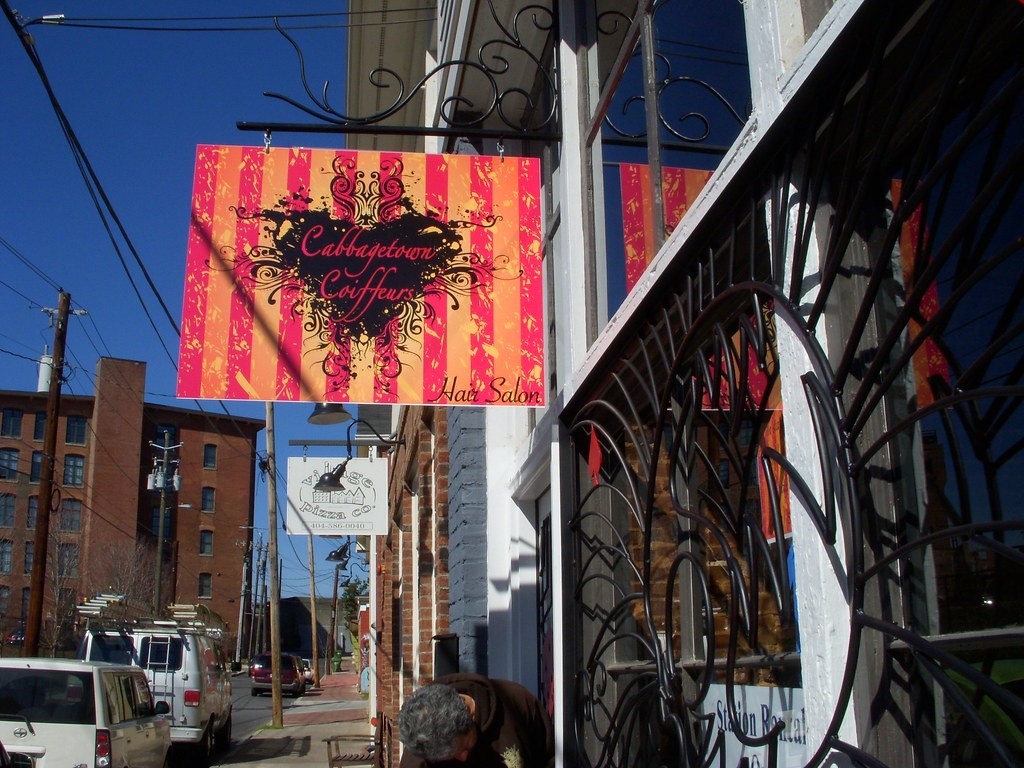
[
  {"xmin": 59, "ymin": 616, "xmax": 241, "ymax": 756},
  {"xmin": 0, "ymin": 656, "xmax": 172, "ymax": 768}
]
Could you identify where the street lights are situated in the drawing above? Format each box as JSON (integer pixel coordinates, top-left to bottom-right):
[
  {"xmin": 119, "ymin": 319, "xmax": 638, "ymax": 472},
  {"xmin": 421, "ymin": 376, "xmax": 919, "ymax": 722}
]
[
  {"xmin": 239, "ymin": 525, "xmax": 263, "ymax": 657},
  {"xmin": 154, "ymin": 504, "xmax": 193, "ymax": 616}
]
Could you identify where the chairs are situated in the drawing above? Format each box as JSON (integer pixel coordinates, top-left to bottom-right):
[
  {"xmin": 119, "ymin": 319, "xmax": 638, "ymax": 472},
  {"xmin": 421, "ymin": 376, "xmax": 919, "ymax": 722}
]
[
  {"xmin": 14, "ymin": 708, "xmax": 49, "ymax": 721},
  {"xmin": 109, "ymin": 650, "xmax": 130, "ymax": 665},
  {"xmin": 323, "ymin": 711, "xmax": 383, "ymax": 768}
]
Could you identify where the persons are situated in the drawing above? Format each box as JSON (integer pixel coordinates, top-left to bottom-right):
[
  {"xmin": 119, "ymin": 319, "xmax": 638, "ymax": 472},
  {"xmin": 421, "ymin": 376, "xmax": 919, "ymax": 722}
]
[{"xmin": 396, "ymin": 672, "xmax": 555, "ymax": 768}]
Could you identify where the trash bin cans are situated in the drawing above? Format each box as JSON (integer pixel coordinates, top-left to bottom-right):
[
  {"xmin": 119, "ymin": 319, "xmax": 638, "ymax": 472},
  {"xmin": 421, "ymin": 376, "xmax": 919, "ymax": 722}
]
[
  {"xmin": 336, "ymin": 650, "xmax": 341, "ymax": 658},
  {"xmin": 333, "ymin": 659, "xmax": 341, "ymax": 672}
]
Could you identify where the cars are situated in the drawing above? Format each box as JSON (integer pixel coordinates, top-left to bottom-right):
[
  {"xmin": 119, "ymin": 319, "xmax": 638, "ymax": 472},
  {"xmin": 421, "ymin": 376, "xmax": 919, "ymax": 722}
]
[
  {"xmin": 301, "ymin": 658, "xmax": 315, "ymax": 684},
  {"xmin": 4, "ymin": 629, "xmax": 27, "ymax": 647},
  {"xmin": 248, "ymin": 658, "xmax": 253, "ymax": 677}
]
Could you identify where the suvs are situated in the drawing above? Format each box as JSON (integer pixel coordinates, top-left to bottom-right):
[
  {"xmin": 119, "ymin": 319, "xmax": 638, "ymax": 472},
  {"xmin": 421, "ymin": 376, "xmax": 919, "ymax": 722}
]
[{"xmin": 250, "ymin": 651, "xmax": 306, "ymax": 698}]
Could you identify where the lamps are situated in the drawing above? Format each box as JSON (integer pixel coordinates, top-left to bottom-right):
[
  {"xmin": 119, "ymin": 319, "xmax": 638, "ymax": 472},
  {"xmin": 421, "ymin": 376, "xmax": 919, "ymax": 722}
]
[
  {"xmin": 307, "ymin": 403, "xmax": 354, "ymax": 424},
  {"xmin": 314, "ymin": 418, "xmax": 406, "ymax": 490},
  {"xmin": 325, "ymin": 540, "xmax": 369, "ymax": 587}
]
